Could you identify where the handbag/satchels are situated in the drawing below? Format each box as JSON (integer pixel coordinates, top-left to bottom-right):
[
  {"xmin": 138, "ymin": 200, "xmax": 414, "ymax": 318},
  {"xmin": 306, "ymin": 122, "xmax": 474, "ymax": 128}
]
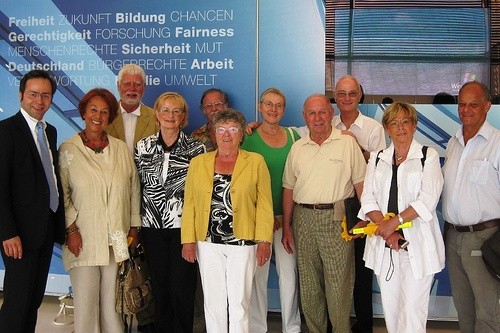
[{"xmin": 116, "ymin": 244, "xmax": 155, "ymax": 314}]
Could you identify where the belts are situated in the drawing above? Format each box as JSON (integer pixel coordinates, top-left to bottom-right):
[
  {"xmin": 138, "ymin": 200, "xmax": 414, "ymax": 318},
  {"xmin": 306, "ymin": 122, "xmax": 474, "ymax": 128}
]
[
  {"xmin": 446, "ymin": 219, "xmax": 500, "ymax": 232},
  {"xmin": 299, "ymin": 204, "xmax": 334, "ymax": 211}
]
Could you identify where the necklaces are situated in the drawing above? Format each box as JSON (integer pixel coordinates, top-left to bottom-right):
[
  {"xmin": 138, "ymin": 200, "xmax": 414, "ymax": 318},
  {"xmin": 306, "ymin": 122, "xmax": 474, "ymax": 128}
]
[
  {"xmin": 394, "ymin": 149, "xmax": 408, "ymax": 161},
  {"xmin": 261, "ymin": 125, "xmax": 280, "ymax": 137}
]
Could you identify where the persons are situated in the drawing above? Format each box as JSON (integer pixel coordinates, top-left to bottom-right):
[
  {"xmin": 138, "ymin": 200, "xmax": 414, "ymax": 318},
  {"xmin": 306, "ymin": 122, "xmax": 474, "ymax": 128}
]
[
  {"xmin": 356, "ymin": 101, "xmax": 446, "ymax": 333},
  {"xmin": 179, "ymin": 107, "xmax": 274, "ymax": 333},
  {"xmin": 0, "ymin": 69, "xmax": 67, "ymax": 333},
  {"xmin": 440, "ymin": 80, "xmax": 500, "ymax": 333},
  {"xmin": 281, "ymin": 94, "xmax": 366, "ymax": 333},
  {"xmin": 239, "ymin": 88, "xmax": 304, "ymax": 333},
  {"xmin": 128, "ymin": 91, "xmax": 209, "ymax": 333},
  {"xmin": 102, "ymin": 64, "xmax": 163, "ymax": 332},
  {"xmin": 57, "ymin": 87, "xmax": 143, "ymax": 333},
  {"xmin": 244, "ymin": 76, "xmax": 387, "ymax": 333},
  {"xmin": 189, "ymin": 89, "xmax": 263, "ymax": 333}
]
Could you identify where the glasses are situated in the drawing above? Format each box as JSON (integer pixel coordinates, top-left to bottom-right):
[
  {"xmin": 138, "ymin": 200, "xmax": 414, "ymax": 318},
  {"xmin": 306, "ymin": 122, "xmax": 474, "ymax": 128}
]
[
  {"xmin": 158, "ymin": 107, "xmax": 184, "ymax": 114},
  {"xmin": 261, "ymin": 101, "xmax": 285, "ymax": 109},
  {"xmin": 202, "ymin": 101, "xmax": 225, "ymax": 109},
  {"xmin": 334, "ymin": 90, "xmax": 359, "ymax": 97},
  {"xmin": 386, "ymin": 118, "xmax": 413, "ymax": 128},
  {"xmin": 215, "ymin": 127, "xmax": 242, "ymax": 135}
]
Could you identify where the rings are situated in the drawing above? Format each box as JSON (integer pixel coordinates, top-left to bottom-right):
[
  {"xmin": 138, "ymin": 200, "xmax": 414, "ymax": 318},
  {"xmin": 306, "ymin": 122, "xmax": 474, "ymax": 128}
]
[{"xmin": 265, "ymin": 258, "xmax": 269, "ymax": 261}]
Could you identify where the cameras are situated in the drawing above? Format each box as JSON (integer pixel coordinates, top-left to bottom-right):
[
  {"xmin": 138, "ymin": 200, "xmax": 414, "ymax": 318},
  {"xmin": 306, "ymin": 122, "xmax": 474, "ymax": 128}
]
[{"xmin": 397, "ymin": 239, "xmax": 409, "ymax": 248}]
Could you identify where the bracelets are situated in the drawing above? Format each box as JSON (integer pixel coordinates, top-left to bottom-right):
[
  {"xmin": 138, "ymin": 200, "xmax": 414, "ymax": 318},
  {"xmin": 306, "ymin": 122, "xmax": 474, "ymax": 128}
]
[
  {"xmin": 65, "ymin": 226, "xmax": 79, "ymax": 237},
  {"xmin": 397, "ymin": 214, "xmax": 404, "ymax": 226}
]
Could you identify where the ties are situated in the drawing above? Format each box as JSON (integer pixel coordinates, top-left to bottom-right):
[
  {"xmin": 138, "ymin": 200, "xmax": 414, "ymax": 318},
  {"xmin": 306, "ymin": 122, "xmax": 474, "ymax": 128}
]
[{"xmin": 36, "ymin": 122, "xmax": 59, "ymax": 213}]
